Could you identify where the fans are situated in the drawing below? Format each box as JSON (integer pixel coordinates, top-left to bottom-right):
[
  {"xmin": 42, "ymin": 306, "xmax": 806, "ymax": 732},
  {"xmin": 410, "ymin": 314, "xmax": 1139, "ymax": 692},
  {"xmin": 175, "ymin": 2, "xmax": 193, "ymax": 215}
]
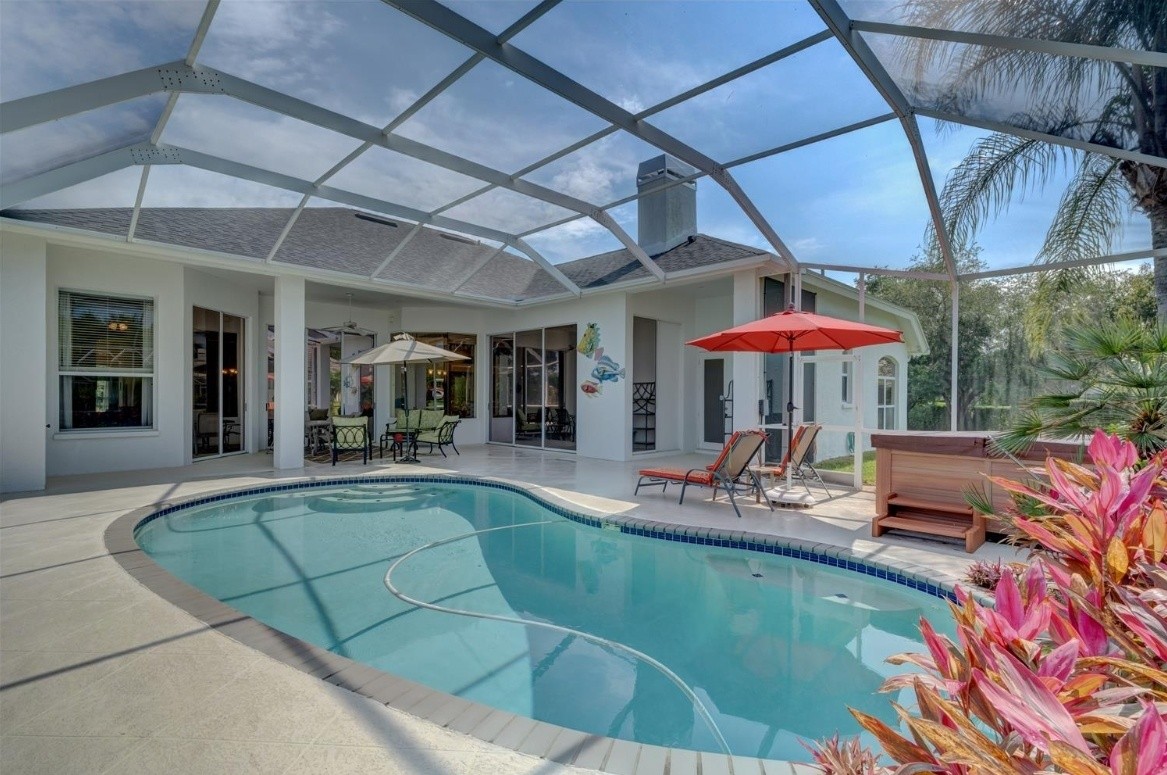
[{"xmin": 323, "ymin": 294, "xmax": 376, "ymax": 338}]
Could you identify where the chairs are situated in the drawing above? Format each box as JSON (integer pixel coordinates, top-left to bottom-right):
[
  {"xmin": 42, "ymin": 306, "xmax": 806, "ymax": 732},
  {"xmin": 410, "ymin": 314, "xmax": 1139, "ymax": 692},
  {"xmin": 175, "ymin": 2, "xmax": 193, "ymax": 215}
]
[
  {"xmin": 706, "ymin": 425, "xmax": 832, "ymax": 503},
  {"xmin": 308, "ymin": 409, "xmax": 373, "ymax": 466},
  {"xmin": 635, "ymin": 431, "xmax": 775, "ymax": 517},
  {"xmin": 386, "ymin": 409, "xmax": 461, "ymax": 458},
  {"xmin": 506, "ymin": 407, "xmax": 575, "ymax": 443},
  {"xmin": 194, "ymin": 412, "xmax": 240, "ymax": 452}
]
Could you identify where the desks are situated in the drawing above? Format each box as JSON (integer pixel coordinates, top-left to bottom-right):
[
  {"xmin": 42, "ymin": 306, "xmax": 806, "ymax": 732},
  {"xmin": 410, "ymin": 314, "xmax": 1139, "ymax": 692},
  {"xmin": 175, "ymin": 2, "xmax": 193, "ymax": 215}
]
[
  {"xmin": 305, "ymin": 419, "xmax": 333, "ymax": 459},
  {"xmin": 379, "ymin": 434, "xmax": 416, "ymax": 461},
  {"xmin": 711, "ymin": 466, "xmax": 781, "ymax": 502}
]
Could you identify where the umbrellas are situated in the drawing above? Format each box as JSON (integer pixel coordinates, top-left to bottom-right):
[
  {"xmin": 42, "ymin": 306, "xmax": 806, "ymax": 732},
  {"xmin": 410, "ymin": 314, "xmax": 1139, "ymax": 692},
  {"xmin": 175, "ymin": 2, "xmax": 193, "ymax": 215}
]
[
  {"xmin": 683, "ymin": 311, "xmax": 905, "ymax": 462},
  {"xmin": 335, "ymin": 334, "xmax": 471, "ymax": 458}
]
